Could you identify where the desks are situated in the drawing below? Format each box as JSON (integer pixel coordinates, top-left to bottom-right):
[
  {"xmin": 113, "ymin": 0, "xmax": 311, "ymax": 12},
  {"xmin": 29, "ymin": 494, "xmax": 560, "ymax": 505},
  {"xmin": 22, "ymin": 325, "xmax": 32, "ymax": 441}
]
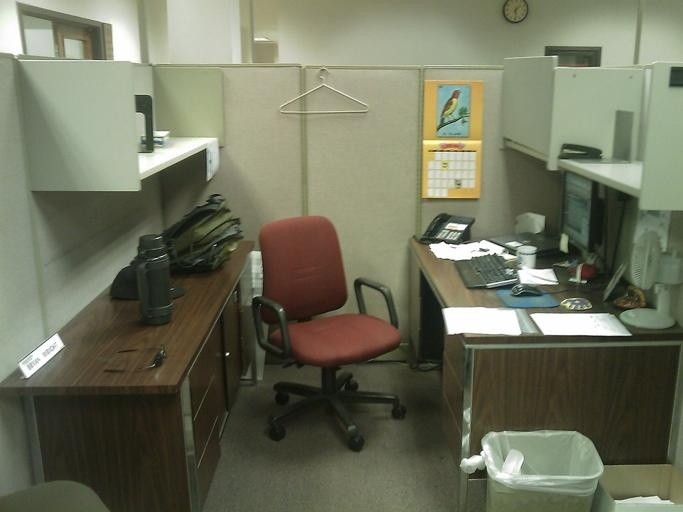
[{"xmin": 407, "ymin": 229, "xmax": 683, "ymax": 512}]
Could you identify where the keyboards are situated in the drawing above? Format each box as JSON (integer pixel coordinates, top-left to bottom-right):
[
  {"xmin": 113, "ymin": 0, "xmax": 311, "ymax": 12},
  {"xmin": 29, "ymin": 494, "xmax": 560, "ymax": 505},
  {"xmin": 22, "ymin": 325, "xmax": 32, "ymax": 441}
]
[{"xmin": 455, "ymin": 253, "xmax": 520, "ymax": 289}]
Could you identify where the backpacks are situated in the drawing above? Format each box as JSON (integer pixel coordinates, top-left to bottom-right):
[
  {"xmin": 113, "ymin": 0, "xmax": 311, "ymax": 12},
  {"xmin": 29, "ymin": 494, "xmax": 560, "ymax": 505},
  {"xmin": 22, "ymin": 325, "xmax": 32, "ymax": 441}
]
[{"xmin": 164, "ymin": 192, "xmax": 244, "ymax": 274}]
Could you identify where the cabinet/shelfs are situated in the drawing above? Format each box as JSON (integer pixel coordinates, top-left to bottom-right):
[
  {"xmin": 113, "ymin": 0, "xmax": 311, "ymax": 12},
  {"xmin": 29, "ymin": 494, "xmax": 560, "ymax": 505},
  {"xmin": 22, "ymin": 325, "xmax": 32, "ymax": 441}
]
[{"xmin": 0, "ymin": 237, "xmax": 258, "ymax": 511}]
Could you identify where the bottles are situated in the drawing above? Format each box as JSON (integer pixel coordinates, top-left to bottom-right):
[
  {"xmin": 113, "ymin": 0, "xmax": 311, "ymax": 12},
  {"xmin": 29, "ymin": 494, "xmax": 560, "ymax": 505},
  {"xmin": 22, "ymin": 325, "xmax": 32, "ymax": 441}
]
[{"xmin": 134, "ymin": 234, "xmax": 173, "ymax": 323}]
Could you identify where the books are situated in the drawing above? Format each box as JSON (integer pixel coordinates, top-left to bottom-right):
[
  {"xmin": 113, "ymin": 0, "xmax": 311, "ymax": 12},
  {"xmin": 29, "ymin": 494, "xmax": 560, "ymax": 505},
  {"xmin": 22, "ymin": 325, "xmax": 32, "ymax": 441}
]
[{"xmin": 518, "ymin": 267, "xmax": 559, "ymax": 285}]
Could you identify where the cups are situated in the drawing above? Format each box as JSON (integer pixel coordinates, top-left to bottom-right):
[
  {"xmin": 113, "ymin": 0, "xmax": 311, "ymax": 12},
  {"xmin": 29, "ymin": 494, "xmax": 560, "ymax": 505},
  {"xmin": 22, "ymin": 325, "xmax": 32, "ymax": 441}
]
[{"xmin": 519, "ymin": 244, "xmax": 536, "ymax": 270}]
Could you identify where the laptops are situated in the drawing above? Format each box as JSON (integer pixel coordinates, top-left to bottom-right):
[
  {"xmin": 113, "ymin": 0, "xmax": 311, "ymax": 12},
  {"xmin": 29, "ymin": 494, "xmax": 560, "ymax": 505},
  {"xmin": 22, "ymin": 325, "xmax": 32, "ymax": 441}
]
[{"xmin": 486, "ymin": 231, "xmax": 561, "ymax": 258}]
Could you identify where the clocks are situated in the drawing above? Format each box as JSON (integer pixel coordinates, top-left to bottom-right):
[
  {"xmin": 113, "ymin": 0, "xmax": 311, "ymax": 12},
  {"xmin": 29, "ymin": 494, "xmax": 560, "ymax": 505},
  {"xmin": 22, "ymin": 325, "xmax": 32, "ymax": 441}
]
[{"xmin": 502, "ymin": 0, "xmax": 529, "ymax": 24}]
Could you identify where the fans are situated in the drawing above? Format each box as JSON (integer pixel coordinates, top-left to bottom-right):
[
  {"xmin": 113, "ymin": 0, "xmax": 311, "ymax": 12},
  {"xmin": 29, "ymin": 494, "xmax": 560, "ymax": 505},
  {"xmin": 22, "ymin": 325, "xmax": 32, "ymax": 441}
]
[{"xmin": 619, "ymin": 229, "xmax": 682, "ymax": 330}]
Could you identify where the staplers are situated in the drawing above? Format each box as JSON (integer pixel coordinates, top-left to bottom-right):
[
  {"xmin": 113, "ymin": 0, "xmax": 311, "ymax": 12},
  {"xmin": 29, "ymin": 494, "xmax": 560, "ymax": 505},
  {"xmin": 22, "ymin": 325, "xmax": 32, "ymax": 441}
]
[{"xmin": 558, "ymin": 143, "xmax": 604, "ymax": 160}]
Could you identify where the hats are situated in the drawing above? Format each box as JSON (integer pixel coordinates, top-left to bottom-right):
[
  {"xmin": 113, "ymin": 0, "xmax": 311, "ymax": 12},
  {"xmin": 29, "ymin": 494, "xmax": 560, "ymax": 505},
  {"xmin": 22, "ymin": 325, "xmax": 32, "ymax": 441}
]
[{"xmin": 109, "ymin": 265, "xmax": 186, "ymax": 303}]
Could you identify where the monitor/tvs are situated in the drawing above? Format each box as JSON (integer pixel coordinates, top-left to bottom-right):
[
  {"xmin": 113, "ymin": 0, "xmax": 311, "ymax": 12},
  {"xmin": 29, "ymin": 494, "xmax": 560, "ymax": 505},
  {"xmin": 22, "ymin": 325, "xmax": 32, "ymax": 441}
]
[{"xmin": 554, "ymin": 168, "xmax": 604, "ymax": 268}]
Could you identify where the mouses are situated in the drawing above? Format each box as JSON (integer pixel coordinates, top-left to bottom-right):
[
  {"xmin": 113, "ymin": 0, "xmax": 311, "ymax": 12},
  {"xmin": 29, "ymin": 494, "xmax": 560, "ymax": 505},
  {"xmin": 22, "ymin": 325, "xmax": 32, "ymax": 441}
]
[{"xmin": 512, "ymin": 284, "xmax": 542, "ymax": 296}]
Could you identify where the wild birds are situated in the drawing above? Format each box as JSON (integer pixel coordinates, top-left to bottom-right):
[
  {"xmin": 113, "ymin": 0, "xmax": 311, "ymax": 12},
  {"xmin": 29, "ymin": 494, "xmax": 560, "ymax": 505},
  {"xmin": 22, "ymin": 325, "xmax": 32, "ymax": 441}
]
[{"xmin": 440, "ymin": 89, "xmax": 462, "ymax": 126}]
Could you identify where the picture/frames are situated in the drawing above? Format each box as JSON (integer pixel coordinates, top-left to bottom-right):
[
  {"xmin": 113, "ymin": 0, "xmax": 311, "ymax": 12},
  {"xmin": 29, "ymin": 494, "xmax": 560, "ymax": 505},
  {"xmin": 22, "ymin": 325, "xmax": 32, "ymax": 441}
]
[{"xmin": 545, "ymin": 45, "xmax": 602, "ymax": 68}]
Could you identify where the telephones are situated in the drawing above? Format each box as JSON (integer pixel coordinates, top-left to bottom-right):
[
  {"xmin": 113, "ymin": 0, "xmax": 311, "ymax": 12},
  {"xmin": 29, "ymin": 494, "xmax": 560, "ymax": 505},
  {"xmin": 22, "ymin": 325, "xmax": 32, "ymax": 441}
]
[{"xmin": 420, "ymin": 213, "xmax": 475, "ymax": 244}]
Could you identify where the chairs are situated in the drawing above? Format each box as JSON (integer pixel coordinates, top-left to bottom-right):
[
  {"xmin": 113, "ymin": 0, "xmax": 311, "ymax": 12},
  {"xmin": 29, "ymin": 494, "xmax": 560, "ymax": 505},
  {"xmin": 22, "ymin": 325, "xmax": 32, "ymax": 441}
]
[
  {"xmin": 0, "ymin": 479, "xmax": 113, "ymax": 511},
  {"xmin": 251, "ymin": 215, "xmax": 407, "ymax": 451}
]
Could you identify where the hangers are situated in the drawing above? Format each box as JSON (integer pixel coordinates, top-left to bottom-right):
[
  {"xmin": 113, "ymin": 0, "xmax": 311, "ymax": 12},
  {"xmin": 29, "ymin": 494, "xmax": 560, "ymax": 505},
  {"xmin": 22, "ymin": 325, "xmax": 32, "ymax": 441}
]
[{"xmin": 278, "ymin": 67, "xmax": 369, "ymax": 115}]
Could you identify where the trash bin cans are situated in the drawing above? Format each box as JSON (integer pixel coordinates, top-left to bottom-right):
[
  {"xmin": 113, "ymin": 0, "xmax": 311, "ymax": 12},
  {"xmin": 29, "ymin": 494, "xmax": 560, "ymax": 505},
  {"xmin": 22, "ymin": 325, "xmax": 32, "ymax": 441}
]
[{"xmin": 480, "ymin": 430, "xmax": 604, "ymax": 512}]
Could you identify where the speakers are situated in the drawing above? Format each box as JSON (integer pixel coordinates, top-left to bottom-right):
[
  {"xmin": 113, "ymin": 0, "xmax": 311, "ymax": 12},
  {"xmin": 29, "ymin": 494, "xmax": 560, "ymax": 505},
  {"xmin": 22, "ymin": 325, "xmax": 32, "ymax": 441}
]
[{"xmin": 611, "ymin": 109, "xmax": 640, "ymax": 163}]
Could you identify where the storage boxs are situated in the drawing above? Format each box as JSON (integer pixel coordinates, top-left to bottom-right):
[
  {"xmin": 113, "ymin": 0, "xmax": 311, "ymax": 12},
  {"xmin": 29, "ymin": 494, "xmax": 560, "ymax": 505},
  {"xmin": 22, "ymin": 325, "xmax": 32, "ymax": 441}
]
[{"xmin": 593, "ymin": 461, "xmax": 683, "ymax": 512}]
